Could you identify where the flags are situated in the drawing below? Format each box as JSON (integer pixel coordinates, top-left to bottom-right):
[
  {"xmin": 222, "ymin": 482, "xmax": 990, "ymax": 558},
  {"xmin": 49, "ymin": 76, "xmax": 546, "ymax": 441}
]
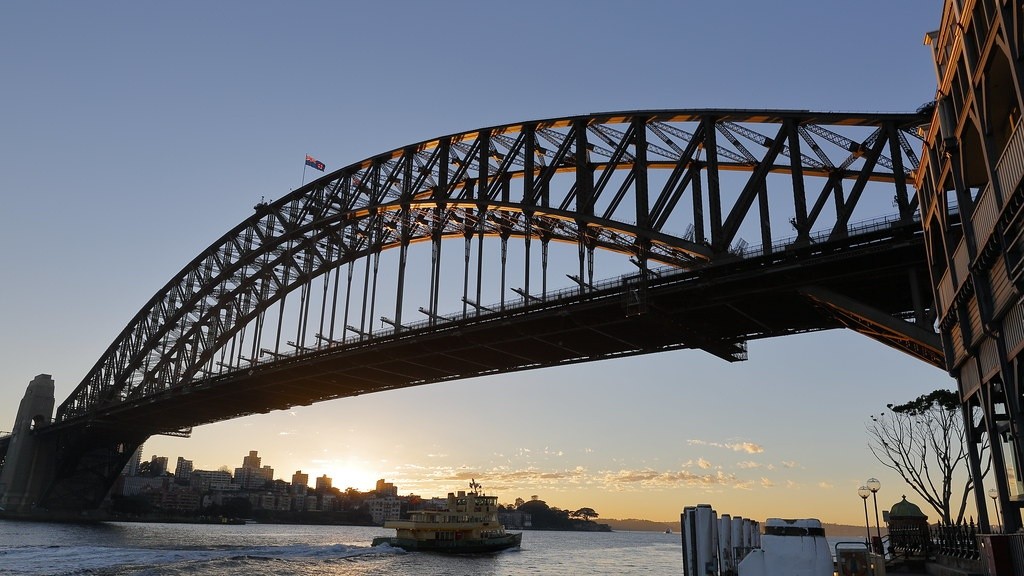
[{"xmin": 305, "ymin": 155, "xmax": 325, "ymax": 172}]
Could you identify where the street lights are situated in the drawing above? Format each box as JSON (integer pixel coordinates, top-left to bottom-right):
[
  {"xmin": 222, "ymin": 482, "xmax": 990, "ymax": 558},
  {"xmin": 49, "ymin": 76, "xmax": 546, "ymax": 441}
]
[
  {"xmin": 988, "ymin": 489, "xmax": 1002, "ymax": 534},
  {"xmin": 867, "ymin": 478, "xmax": 883, "ymax": 556},
  {"xmin": 857, "ymin": 485, "xmax": 872, "ymax": 553}
]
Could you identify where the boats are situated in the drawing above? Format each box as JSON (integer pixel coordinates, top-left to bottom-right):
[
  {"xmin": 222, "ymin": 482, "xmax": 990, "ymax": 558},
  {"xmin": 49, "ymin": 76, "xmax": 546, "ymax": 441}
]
[{"xmin": 372, "ymin": 487, "xmax": 523, "ymax": 553}]
